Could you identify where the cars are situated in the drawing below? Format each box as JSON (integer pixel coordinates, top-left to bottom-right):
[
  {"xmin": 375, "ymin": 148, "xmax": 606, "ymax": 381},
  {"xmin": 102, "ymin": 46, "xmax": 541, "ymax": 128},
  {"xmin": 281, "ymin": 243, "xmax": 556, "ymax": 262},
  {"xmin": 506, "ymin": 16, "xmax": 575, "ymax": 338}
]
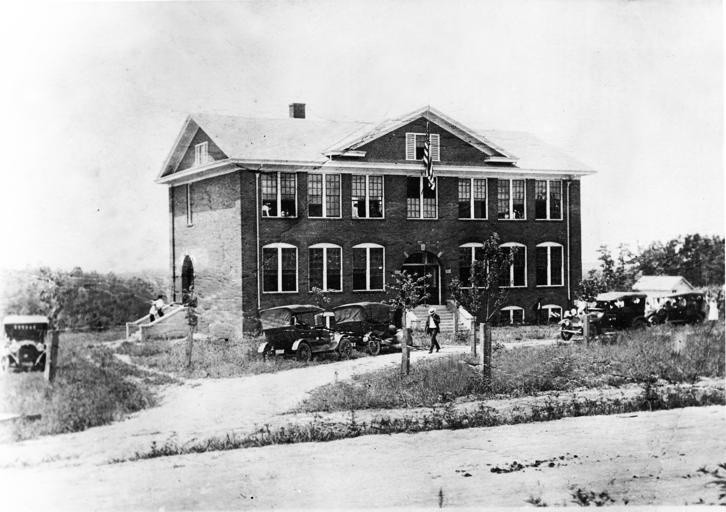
[
  {"xmin": 258, "ymin": 304, "xmax": 354, "ymax": 362},
  {"xmin": 1, "ymin": 314, "xmax": 53, "ymax": 375},
  {"xmin": 558, "ymin": 290, "xmax": 651, "ymax": 342},
  {"xmin": 333, "ymin": 302, "xmax": 414, "ymax": 356},
  {"xmin": 647, "ymin": 290, "xmax": 708, "ymax": 328}
]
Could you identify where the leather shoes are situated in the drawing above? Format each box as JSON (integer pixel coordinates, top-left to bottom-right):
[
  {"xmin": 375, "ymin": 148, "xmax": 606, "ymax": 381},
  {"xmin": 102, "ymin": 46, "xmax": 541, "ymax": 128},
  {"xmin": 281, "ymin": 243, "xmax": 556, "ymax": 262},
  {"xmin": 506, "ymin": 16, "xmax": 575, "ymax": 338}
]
[
  {"xmin": 436, "ymin": 348, "xmax": 441, "ymax": 352},
  {"xmin": 428, "ymin": 351, "xmax": 433, "ymax": 354}
]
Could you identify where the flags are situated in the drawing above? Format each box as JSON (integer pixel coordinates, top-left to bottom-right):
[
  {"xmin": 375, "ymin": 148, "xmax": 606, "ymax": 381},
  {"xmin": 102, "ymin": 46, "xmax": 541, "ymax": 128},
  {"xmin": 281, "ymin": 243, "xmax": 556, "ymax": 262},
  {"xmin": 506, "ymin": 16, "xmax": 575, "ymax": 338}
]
[{"xmin": 421, "ymin": 120, "xmax": 436, "ymax": 190}]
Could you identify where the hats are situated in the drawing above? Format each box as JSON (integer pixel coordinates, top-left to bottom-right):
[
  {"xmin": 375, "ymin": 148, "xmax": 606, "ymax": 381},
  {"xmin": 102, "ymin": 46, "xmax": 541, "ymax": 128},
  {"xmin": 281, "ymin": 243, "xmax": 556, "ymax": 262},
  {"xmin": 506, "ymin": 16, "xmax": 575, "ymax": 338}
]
[{"xmin": 428, "ymin": 307, "xmax": 436, "ymax": 314}]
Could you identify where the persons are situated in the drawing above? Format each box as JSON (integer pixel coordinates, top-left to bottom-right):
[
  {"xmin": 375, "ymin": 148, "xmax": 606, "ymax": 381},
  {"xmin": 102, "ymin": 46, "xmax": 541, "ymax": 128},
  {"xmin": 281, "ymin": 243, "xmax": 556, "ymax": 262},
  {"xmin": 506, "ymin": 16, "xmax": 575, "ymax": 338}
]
[
  {"xmin": 353, "ymin": 204, "xmax": 359, "ymax": 217},
  {"xmin": 707, "ymin": 293, "xmax": 721, "ymax": 328},
  {"xmin": 262, "ymin": 202, "xmax": 272, "ymax": 216},
  {"xmin": 426, "ymin": 308, "xmax": 440, "ymax": 353},
  {"xmin": 149, "ymin": 295, "xmax": 164, "ymax": 322},
  {"xmin": 281, "ymin": 209, "xmax": 290, "ymax": 217}
]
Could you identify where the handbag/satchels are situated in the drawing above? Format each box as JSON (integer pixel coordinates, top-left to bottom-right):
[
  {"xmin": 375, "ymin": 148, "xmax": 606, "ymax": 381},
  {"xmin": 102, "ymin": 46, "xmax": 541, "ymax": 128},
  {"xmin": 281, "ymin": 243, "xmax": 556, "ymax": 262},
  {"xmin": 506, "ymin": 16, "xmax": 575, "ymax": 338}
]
[{"xmin": 426, "ymin": 326, "xmax": 429, "ymax": 334}]
